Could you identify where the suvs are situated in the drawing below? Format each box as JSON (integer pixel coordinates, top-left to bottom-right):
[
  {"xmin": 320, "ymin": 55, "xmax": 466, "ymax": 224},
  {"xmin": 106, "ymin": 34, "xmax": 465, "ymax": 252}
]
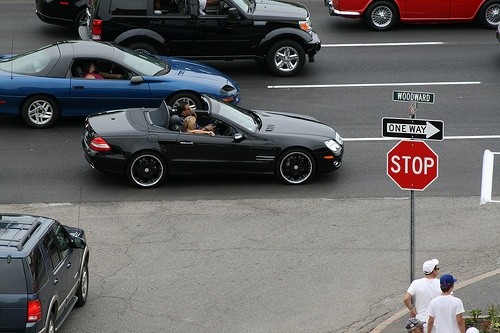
[
  {"xmin": 77, "ymin": 0, "xmax": 321, "ymax": 77},
  {"xmin": 0, "ymin": 212, "xmax": 90, "ymax": 333}
]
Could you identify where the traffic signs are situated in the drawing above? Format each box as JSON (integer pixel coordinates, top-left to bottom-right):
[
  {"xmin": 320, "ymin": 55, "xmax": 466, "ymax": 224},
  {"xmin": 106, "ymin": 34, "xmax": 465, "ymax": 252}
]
[{"xmin": 381, "ymin": 116, "xmax": 445, "ymax": 142}]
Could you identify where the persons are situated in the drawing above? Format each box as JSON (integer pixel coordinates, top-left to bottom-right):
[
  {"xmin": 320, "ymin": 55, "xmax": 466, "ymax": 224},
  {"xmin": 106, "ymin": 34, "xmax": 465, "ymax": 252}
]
[
  {"xmin": 406, "ymin": 318, "xmax": 426, "ymax": 333},
  {"xmin": 426, "ymin": 274, "xmax": 465, "ymax": 333},
  {"xmin": 84, "ymin": 61, "xmax": 122, "ymax": 79},
  {"xmin": 404, "ymin": 259, "xmax": 453, "ymax": 333},
  {"xmin": 177, "ymin": 101, "xmax": 216, "ymax": 136}
]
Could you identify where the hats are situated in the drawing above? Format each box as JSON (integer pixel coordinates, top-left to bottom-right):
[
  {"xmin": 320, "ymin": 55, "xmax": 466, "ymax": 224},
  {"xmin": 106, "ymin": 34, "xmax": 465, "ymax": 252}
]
[
  {"xmin": 440, "ymin": 274, "xmax": 457, "ymax": 283},
  {"xmin": 405, "ymin": 318, "xmax": 426, "ymax": 333},
  {"xmin": 423, "ymin": 258, "xmax": 439, "ymax": 274}
]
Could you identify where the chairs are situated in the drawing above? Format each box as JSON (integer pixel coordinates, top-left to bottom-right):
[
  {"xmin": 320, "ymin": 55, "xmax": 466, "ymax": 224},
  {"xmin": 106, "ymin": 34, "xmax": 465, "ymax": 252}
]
[
  {"xmin": 168, "ymin": 106, "xmax": 184, "ymax": 133},
  {"xmin": 72, "ymin": 62, "xmax": 87, "ymax": 77}
]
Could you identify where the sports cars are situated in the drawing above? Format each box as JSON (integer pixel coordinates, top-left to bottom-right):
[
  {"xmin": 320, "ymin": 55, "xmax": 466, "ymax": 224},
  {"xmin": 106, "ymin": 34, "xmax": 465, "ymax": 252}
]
[
  {"xmin": 82, "ymin": 95, "xmax": 344, "ymax": 189},
  {"xmin": 0, "ymin": 39, "xmax": 240, "ymax": 130}
]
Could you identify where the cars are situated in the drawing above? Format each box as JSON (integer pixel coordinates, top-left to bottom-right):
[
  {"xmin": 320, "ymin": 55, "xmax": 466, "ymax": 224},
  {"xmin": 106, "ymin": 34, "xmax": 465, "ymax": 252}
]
[{"xmin": 323, "ymin": 0, "xmax": 500, "ymax": 31}]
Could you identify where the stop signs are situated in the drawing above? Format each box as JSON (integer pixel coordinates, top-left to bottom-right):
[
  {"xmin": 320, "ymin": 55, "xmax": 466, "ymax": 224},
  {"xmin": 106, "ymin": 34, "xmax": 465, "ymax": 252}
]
[{"xmin": 386, "ymin": 140, "xmax": 438, "ymax": 192}]
